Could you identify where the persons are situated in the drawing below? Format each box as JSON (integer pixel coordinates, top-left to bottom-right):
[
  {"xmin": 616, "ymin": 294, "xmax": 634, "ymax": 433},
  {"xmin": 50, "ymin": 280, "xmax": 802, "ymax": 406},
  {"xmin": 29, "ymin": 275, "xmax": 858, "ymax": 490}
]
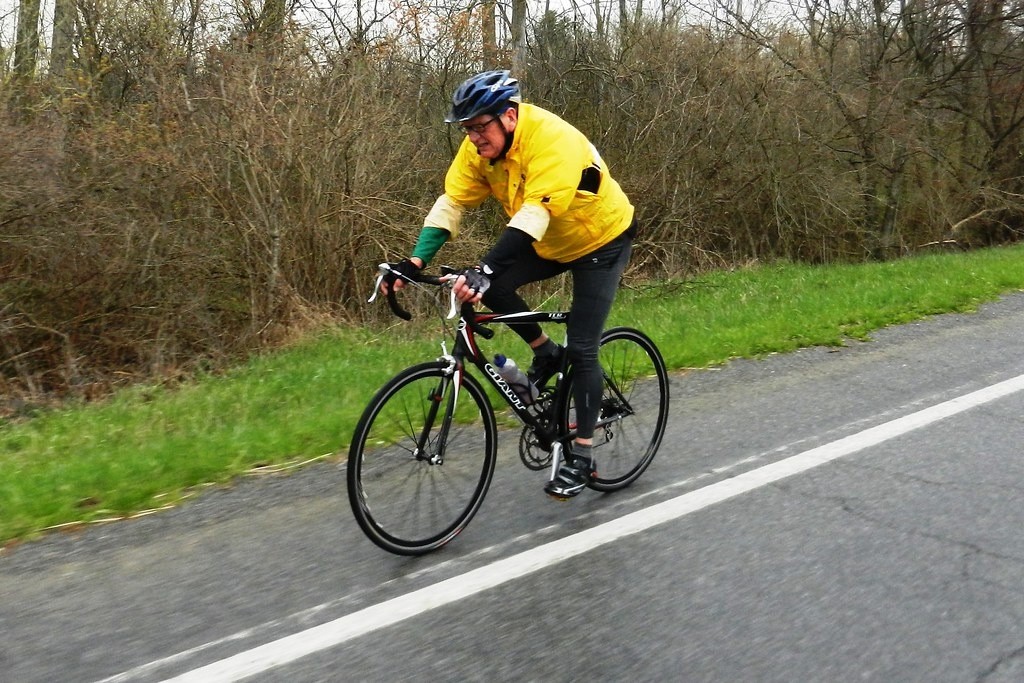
[{"xmin": 381, "ymin": 69, "xmax": 637, "ymax": 498}]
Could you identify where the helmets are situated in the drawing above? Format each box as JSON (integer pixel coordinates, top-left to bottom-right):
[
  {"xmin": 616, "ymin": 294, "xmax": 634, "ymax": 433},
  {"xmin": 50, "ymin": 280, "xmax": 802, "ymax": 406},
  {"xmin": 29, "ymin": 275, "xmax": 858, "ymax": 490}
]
[{"xmin": 444, "ymin": 69, "xmax": 521, "ymax": 123}]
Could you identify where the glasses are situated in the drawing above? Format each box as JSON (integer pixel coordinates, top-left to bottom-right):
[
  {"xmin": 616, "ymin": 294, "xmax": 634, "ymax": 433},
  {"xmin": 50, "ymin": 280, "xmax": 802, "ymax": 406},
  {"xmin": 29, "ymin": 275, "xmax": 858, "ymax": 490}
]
[{"xmin": 458, "ymin": 112, "xmax": 506, "ymax": 134}]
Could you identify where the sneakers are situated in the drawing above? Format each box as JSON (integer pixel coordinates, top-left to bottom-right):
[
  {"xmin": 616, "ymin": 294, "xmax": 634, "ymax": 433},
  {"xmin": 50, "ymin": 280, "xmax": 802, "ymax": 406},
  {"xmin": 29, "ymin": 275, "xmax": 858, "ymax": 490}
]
[
  {"xmin": 526, "ymin": 344, "xmax": 565, "ymax": 391},
  {"xmin": 543, "ymin": 454, "xmax": 597, "ymax": 498}
]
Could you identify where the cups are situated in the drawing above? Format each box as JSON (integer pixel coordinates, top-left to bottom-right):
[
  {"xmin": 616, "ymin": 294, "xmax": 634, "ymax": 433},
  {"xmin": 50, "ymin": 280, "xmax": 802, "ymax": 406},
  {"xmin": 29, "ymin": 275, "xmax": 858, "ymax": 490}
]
[{"xmin": 494, "ymin": 353, "xmax": 540, "ymax": 405}]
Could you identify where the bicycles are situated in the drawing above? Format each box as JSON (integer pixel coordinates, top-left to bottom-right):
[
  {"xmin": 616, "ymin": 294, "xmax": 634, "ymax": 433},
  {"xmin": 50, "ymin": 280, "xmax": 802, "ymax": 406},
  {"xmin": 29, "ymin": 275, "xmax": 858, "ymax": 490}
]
[{"xmin": 346, "ymin": 259, "xmax": 671, "ymax": 558}]
